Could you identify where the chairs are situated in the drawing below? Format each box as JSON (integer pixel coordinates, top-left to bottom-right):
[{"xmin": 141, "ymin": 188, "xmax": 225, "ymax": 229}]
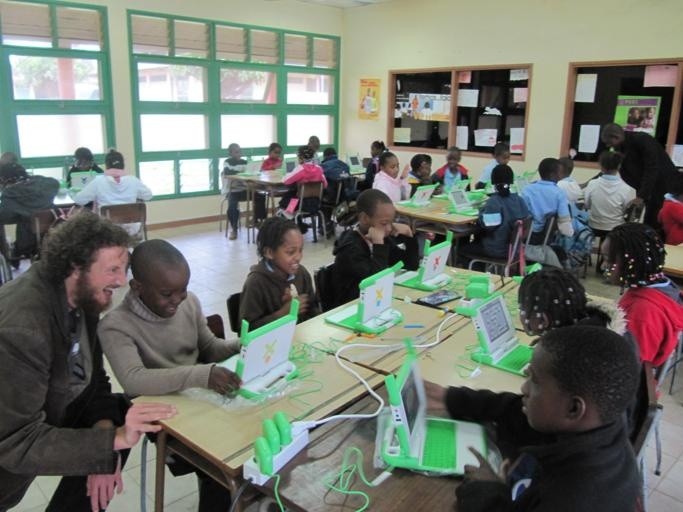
[
  {"xmin": 581, "ymin": 203, "xmax": 646, "ymax": 278},
  {"xmin": 295, "ymin": 181, "xmax": 328, "ymax": 248},
  {"xmin": 226, "ymin": 292, "xmax": 242, "ymax": 337},
  {"xmin": 322, "ymin": 177, "xmax": 358, "ymax": 238},
  {"xmin": 219, "ymin": 172, "xmax": 242, "ymax": 233},
  {"xmin": 523, "ymin": 212, "xmax": 559, "ymax": 262},
  {"xmin": 468, "ymin": 215, "xmax": 534, "ymax": 278},
  {"xmin": 30, "ymin": 208, "xmax": 74, "ymax": 257},
  {"xmin": 631, "ymin": 360, "xmax": 657, "ymax": 512},
  {"xmin": 314, "ymin": 261, "xmax": 335, "ymax": 313},
  {"xmin": 100, "ymin": 203, "xmax": 147, "ymax": 246},
  {"xmin": 140, "ymin": 314, "xmax": 225, "ymax": 511},
  {"xmin": 655, "ymin": 329, "xmax": 680, "ymax": 476}
]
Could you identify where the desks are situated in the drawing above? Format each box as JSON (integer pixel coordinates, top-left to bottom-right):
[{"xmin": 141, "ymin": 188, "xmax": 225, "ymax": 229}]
[
  {"xmin": 503, "ymin": 285, "xmax": 614, "ymax": 332},
  {"xmin": 294, "ymin": 296, "xmax": 473, "ymax": 374},
  {"xmin": 620, "ymin": 244, "xmax": 683, "ymax": 294},
  {"xmin": 52, "ymin": 191, "xmax": 94, "ymax": 209},
  {"xmin": 394, "ymin": 320, "xmax": 540, "ymax": 396},
  {"xmin": 224, "ymin": 170, "xmax": 283, "ymax": 244},
  {"xmin": 393, "ymin": 198, "xmax": 478, "ymax": 266},
  {"xmin": 131, "ymin": 340, "xmax": 386, "ymax": 512},
  {"xmin": 392, "ymin": 266, "xmax": 513, "ymax": 316},
  {"xmin": 250, "ymin": 385, "xmax": 464, "ymax": 512}
]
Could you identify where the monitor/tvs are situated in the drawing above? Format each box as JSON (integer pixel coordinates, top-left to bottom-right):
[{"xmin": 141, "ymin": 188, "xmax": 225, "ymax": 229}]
[
  {"xmin": 523, "ymin": 169, "xmax": 538, "ymax": 182},
  {"xmin": 513, "ymin": 262, "xmax": 543, "ymax": 283},
  {"xmin": 514, "ymin": 176, "xmax": 530, "ymax": 196},
  {"xmin": 69, "ymin": 170, "xmax": 98, "ymax": 193},
  {"xmin": 282, "ymin": 156, "xmax": 298, "ymax": 175},
  {"xmin": 89, "ymin": 169, "xmax": 104, "ymax": 179}
]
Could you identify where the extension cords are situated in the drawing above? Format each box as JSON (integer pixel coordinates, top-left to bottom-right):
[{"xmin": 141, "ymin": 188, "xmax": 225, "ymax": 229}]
[
  {"xmin": 242, "ymin": 424, "xmax": 311, "ymax": 485},
  {"xmin": 459, "ymin": 282, "xmax": 495, "ymax": 309}
]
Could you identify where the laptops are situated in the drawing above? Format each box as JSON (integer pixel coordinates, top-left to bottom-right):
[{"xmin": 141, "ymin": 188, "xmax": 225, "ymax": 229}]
[
  {"xmin": 324, "ymin": 260, "xmax": 405, "ymax": 335},
  {"xmin": 237, "ymin": 157, "xmax": 265, "ymax": 176},
  {"xmin": 448, "ymin": 189, "xmax": 479, "ymax": 216},
  {"xmin": 433, "ymin": 175, "xmax": 473, "ymax": 200},
  {"xmin": 394, "ymin": 231, "xmax": 453, "ymax": 292},
  {"xmin": 215, "ymin": 298, "xmax": 301, "ymax": 402},
  {"xmin": 454, "ymin": 291, "xmax": 535, "ymax": 377},
  {"xmin": 345, "ymin": 152, "xmax": 367, "ymax": 174},
  {"xmin": 397, "ymin": 182, "xmax": 440, "ymax": 208},
  {"xmin": 371, "ymin": 336, "xmax": 488, "ymax": 479}
]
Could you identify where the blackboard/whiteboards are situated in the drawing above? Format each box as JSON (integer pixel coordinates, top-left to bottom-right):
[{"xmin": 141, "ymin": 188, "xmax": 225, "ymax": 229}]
[
  {"xmin": 453, "ymin": 63, "xmax": 532, "ymax": 162},
  {"xmin": 386, "ymin": 68, "xmax": 453, "ymax": 153},
  {"xmin": 671, "ymin": 74, "xmax": 683, "ymax": 170},
  {"xmin": 559, "ymin": 58, "xmax": 679, "ymax": 169}
]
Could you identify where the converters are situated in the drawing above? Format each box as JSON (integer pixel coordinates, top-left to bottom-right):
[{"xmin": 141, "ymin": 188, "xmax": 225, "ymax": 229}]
[
  {"xmin": 273, "ymin": 411, "xmax": 292, "ymax": 446},
  {"xmin": 465, "ymin": 285, "xmax": 489, "ymax": 299},
  {"xmin": 469, "ymin": 275, "xmax": 489, "ymax": 284},
  {"xmin": 261, "ymin": 418, "xmax": 280, "ymax": 455},
  {"xmin": 252, "ymin": 435, "xmax": 275, "ymax": 476}
]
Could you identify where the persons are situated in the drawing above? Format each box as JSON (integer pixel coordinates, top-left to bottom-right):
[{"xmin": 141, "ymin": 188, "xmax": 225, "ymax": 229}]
[
  {"xmin": 658, "ymin": 170, "xmax": 683, "ymax": 246},
  {"xmin": 420, "ymin": 324, "xmax": 644, "ymax": 512},
  {"xmin": 640, "ymin": 107, "xmax": 655, "ymax": 128},
  {"xmin": 584, "ymin": 151, "xmax": 638, "ymax": 237},
  {"xmin": 601, "ymin": 122, "xmax": 677, "ymax": 243},
  {"xmin": 407, "ymin": 154, "xmax": 437, "ymax": 199},
  {"xmin": 97, "ymin": 239, "xmax": 246, "ymax": 512},
  {"xmin": 556, "ymin": 157, "xmax": 583, "ymax": 204},
  {"xmin": 432, "ymin": 147, "xmax": 471, "ymax": 192},
  {"xmin": 600, "ymin": 223, "xmax": 683, "ymax": 369},
  {"xmin": 1, "ymin": 151, "xmax": 16, "ymax": 164},
  {"xmin": 318, "ymin": 147, "xmax": 350, "ymax": 237},
  {"xmin": 308, "ymin": 137, "xmax": 323, "ymax": 164},
  {"xmin": 72, "ymin": 148, "xmax": 153, "ymax": 238},
  {"xmin": 260, "ymin": 142, "xmax": 283, "ymax": 172},
  {"xmin": 516, "ymin": 269, "xmax": 640, "ymax": 440},
  {"xmin": 65, "ymin": 147, "xmax": 103, "ymax": 190},
  {"xmin": 329, "ymin": 191, "xmax": 420, "ymax": 310},
  {"xmin": 519, "ymin": 157, "xmax": 574, "ymax": 246},
  {"xmin": 279, "ymin": 145, "xmax": 328, "ymax": 215},
  {"xmin": 221, "ymin": 142, "xmax": 267, "ymax": 240},
  {"xmin": 456, "ymin": 164, "xmax": 527, "ymax": 273},
  {"xmin": 0, "ymin": 163, "xmax": 60, "ymax": 270},
  {"xmin": 0, "ymin": 207, "xmax": 178, "ymax": 512},
  {"xmin": 474, "ymin": 143, "xmax": 510, "ymax": 190},
  {"xmin": 628, "ymin": 107, "xmax": 645, "ymax": 128},
  {"xmin": 365, "ymin": 140, "xmax": 390, "ymax": 185},
  {"xmin": 239, "ymin": 215, "xmax": 322, "ymax": 328},
  {"xmin": 372, "ymin": 152, "xmax": 411, "ymax": 202}
]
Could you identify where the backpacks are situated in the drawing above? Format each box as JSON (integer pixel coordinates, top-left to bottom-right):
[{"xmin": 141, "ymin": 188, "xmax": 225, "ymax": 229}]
[{"xmin": 556, "ymin": 197, "xmax": 595, "ymax": 269}]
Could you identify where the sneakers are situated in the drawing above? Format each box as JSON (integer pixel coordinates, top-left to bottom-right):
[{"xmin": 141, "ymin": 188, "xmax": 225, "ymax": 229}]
[{"xmin": 230, "ymin": 230, "xmax": 237, "ymax": 239}]
